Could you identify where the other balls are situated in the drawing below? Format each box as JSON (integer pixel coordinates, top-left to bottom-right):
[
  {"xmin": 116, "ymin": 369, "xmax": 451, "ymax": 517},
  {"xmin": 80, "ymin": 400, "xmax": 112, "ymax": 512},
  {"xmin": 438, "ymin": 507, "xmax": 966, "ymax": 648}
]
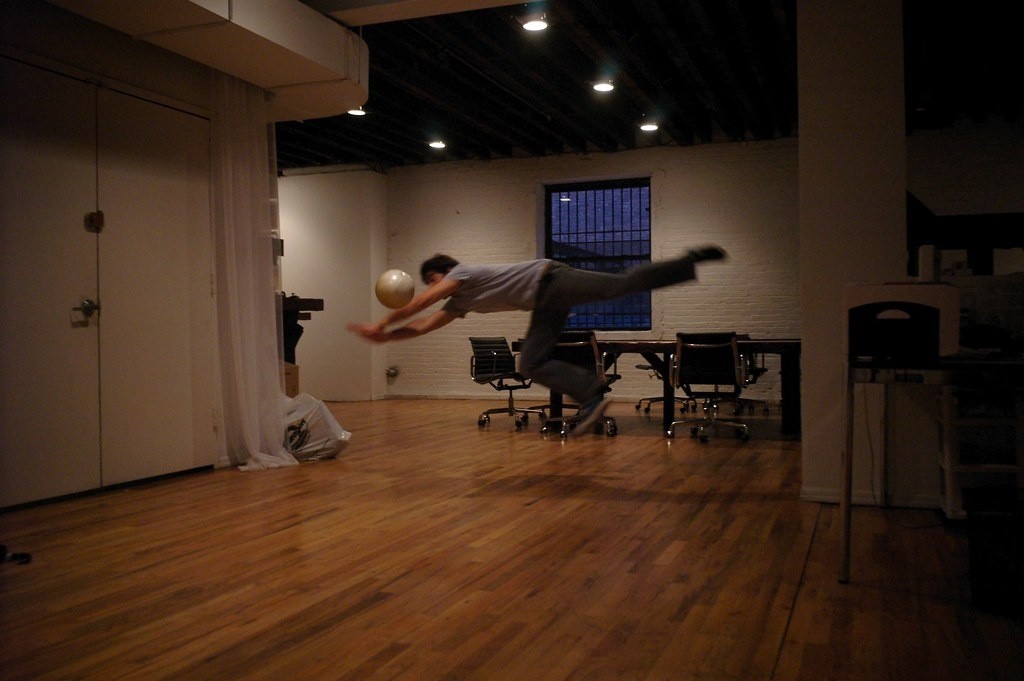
[{"xmin": 374, "ymin": 269, "xmax": 414, "ymax": 308}]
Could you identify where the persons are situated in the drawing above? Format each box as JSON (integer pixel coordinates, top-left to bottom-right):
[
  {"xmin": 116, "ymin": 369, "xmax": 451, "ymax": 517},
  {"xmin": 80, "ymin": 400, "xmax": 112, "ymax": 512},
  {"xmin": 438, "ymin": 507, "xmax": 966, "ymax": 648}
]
[{"xmin": 345, "ymin": 241, "xmax": 732, "ymax": 437}]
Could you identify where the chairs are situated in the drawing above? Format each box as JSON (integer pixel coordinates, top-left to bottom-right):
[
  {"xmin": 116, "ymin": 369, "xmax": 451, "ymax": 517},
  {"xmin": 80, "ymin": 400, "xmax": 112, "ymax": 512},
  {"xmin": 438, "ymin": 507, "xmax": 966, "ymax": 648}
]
[
  {"xmin": 673, "ymin": 330, "xmax": 751, "ymax": 446},
  {"xmin": 540, "ymin": 330, "xmax": 618, "ymax": 439},
  {"xmin": 633, "ymin": 337, "xmax": 700, "ymax": 413},
  {"xmin": 700, "ymin": 331, "xmax": 771, "ymax": 416},
  {"xmin": 467, "ymin": 335, "xmax": 548, "ymax": 432},
  {"xmin": 838, "ymin": 299, "xmax": 953, "ymax": 586}
]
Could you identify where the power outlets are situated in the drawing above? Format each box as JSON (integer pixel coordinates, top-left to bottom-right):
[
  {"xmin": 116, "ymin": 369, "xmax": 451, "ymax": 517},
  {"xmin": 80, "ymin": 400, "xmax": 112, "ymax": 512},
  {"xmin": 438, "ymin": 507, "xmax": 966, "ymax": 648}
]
[{"xmin": 385, "ymin": 365, "xmax": 398, "ymax": 378}]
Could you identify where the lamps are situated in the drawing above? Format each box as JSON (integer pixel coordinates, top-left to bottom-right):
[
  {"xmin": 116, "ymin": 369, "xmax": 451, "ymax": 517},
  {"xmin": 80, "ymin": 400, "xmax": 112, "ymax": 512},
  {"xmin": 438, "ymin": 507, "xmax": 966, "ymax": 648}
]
[
  {"xmin": 639, "ymin": 114, "xmax": 659, "ymax": 134},
  {"xmin": 592, "ymin": 71, "xmax": 615, "ymax": 96},
  {"xmin": 511, "ymin": 0, "xmax": 562, "ymax": 33}
]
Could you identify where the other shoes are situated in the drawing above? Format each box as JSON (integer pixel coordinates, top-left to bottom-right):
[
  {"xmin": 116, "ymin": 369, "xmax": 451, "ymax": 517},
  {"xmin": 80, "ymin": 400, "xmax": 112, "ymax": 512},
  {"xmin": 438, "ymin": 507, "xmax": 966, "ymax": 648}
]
[
  {"xmin": 687, "ymin": 243, "xmax": 726, "ymax": 264},
  {"xmin": 568, "ymin": 396, "xmax": 611, "ymax": 436}
]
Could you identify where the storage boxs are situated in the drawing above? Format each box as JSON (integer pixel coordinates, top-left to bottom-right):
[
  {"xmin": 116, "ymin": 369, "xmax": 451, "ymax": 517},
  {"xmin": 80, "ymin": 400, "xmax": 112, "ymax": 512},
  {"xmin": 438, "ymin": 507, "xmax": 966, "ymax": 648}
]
[{"xmin": 283, "ymin": 360, "xmax": 300, "ymax": 399}]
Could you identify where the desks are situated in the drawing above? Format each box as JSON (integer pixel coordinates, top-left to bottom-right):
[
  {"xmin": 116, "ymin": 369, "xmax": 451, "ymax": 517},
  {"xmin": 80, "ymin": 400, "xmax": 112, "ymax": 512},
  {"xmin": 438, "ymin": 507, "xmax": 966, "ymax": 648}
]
[{"xmin": 510, "ymin": 337, "xmax": 803, "ymax": 443}]
